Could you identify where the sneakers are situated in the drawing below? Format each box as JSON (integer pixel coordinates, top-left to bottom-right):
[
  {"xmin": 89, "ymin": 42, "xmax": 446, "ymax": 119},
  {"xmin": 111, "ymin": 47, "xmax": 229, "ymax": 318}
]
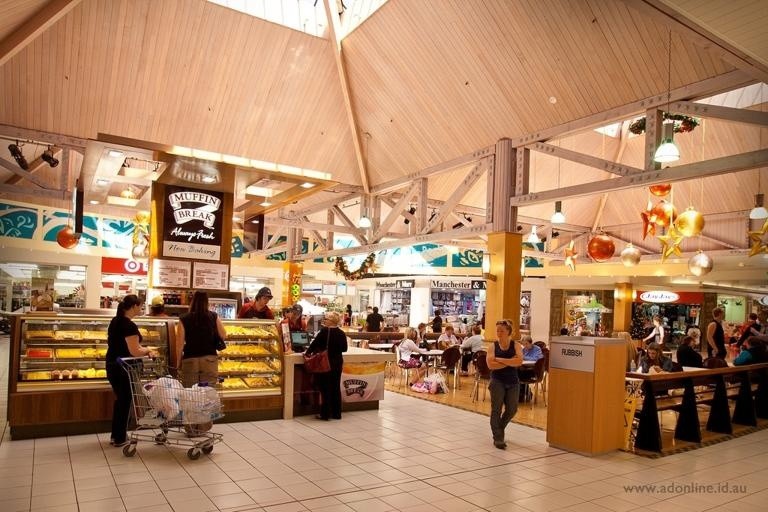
[
  {"xmin": 109, "ymin": 433, "xmax": 130, "ymax": 447},
  {"xmin": 459, "ymin": 370, "xmax": 469, "ymax": 376},
  {"xmin": 124, "ymin": 359, "xmax": 225, "ymax": 426},
  {"xmin": 315, "ymin": 412, "xmax": 341, "ymax": 420},
  {"xmin": 493, "ymin": 437, "xmax": 507, "ymax": 449}
]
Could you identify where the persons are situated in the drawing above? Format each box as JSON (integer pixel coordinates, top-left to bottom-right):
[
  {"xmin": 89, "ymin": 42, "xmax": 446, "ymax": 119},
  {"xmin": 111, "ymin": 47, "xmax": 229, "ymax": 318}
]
[
  {"xmin": 104, "ymin": 293, "xmax": 157, "ymax": 447},
  {"xmin": 281, "ymin": 303, "xmax": 307, "ymax": 332},
  {"xmin": 706, "ymin": 306, "xmax": 727, "ymax": 359},
  {"xmin": 303, "ymin": 311, "xmax": 348, "ymax": 421},
  {"xmin": 363, "ymin": 306, "xmax": 386, "ymax": 332},
  {"xmin": 398, "ymin": 308, "xmax": 486, "ymax": 389},
  {"xmin": 559, "ymin": 327, "xmax": 570, "ymax": 337},
  {"xmin": 635, "ymin": 313, "xmax": 768, "ymax": 373},
  {"xmin": 176, "ymin": 288, "xmax": 228, "ymax": 438},
  {"xmin": 366, "ymin": 305, "xmax": 373, "ymax": 311},
  {"xmin": 234, "ymin": 286, "xmax": 277, "ymax": 321},
  {"xmin": 520, "ymin": 334, "xmax": 544, "ymax": 404},
  {"xmin": 344, "ymin": 304, "xmax": 352, "ymax": 323},
  {"xmin": 485, "ymin": 319, "xmax": 525, "ymax": 450},
  {"xmin": 747, "ymin": 304, "xmax": 767, "ymax": 362}
]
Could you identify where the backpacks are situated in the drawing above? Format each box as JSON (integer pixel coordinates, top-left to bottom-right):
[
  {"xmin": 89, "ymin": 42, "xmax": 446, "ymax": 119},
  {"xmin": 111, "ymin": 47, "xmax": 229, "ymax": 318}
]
[
  {"xmin": 663, "ymin": 361, "xmax": 683, "ymax": 372},
  {"xmin": 705, "ymin": 357, "xmax": 729, "ymax": 368},
  {"xmin": 303, "ymin": 351, "xmax": 331, "ymax": 374},
  {"xmin": 518, "ymin": 383, "xmax": 533, "ymax": 403}
]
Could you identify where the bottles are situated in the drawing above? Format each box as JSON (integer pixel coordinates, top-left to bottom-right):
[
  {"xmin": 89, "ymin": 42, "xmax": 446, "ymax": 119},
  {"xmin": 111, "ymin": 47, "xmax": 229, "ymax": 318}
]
[
  {"xmin": 631, "ymin": 359, "xmax": 637, "ymax": 372},
  {"xmin": 161, "ymin": 290, "xmax": 194, "ymax": 306}
]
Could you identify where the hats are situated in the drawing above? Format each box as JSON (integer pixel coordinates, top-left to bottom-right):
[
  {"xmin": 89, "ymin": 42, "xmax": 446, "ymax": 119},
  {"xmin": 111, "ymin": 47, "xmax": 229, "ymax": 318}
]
[
  {"xmin": 291, "ymin": 304, "xmax": 303, "ymax": 316},
  {"xmin": 151, "ymin": 295, "xmax": 165, "ymax": 307},
  {"xmin": 255, "ymin": 287, "xmax": 273, "ymax": 300}
]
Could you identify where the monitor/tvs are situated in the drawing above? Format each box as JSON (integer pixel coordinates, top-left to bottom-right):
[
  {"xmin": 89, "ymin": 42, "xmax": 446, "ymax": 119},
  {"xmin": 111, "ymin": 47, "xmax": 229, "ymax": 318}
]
[{"xmin": 290, "ymin": 331, "xmax": 310, "ymax": 346}]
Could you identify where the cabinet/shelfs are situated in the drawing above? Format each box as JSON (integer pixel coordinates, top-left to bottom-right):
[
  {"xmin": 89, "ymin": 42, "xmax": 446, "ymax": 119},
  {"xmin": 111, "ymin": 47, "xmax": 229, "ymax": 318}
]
[
  {"xmin": 7, "ymin": 314, "xmax": 173, "ymax": 433},
  {"xmin": 168, "ymin": 319, "xmax": 285, "ymax": 419}
]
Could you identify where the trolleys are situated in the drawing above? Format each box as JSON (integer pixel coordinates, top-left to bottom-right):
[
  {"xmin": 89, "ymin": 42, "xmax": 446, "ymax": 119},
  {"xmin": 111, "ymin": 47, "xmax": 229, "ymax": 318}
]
[{"xmin": 118, "ymin": 354, "xmax": 227, "ymax": 460}]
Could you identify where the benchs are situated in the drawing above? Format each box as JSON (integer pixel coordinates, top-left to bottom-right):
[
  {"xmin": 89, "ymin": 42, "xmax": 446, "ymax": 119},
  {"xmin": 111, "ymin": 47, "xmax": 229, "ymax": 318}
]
[{"xmin": 625, "ymin": 360, "xmax": 768, "ymax": 453}]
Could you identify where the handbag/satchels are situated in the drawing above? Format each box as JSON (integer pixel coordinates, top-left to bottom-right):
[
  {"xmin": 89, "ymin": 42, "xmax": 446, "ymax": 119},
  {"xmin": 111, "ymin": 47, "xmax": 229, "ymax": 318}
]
[
  {"xmin": 733, "ymin": 350, "xmax": 751, "ymax": 366},
  {"xmin": 212, "ymin": 333, "xmax": 226, "ymax": 351},
  {"xmin": 412, "ymin": 373, "xmax": 449, "ymax": 393}
]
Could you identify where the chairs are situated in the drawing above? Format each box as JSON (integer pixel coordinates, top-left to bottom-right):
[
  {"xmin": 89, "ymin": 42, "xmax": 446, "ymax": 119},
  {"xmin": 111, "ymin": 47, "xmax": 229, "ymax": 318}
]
[
  {"xmin": 703, "ymin": 357, "xmax": 726, "ymax": 389},
  {"xmin": 351, "ymin": 330, "xmax": 549, "ymax": 410},
  {"xmin": 650, "ymin": 361, "xmax": 682, "ymax": 428}
]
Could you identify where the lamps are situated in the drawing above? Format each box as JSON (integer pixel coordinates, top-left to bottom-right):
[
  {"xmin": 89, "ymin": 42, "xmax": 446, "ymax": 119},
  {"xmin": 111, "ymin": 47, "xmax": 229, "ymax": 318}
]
[
  {"xmin": 652, "ymin": 26, "xmax": 682, "ymax": 164},
  {"xmin": 358, "ymin": 135, "xmax": 371, "ymax": 229},
  {"xmin": 5, "ymin": 138, "xmax": 59, "ymax": 172},
  {"xmin": 402, "ymin": 203, "xmax": 472, "ymax": 230},
  {"xmin": 551, "ymin": 136, "xmax": 568, "ymax": 224},
  {"xmin": 749, "ymin": 81, "xmax": 767, "ymax": 221}
]
